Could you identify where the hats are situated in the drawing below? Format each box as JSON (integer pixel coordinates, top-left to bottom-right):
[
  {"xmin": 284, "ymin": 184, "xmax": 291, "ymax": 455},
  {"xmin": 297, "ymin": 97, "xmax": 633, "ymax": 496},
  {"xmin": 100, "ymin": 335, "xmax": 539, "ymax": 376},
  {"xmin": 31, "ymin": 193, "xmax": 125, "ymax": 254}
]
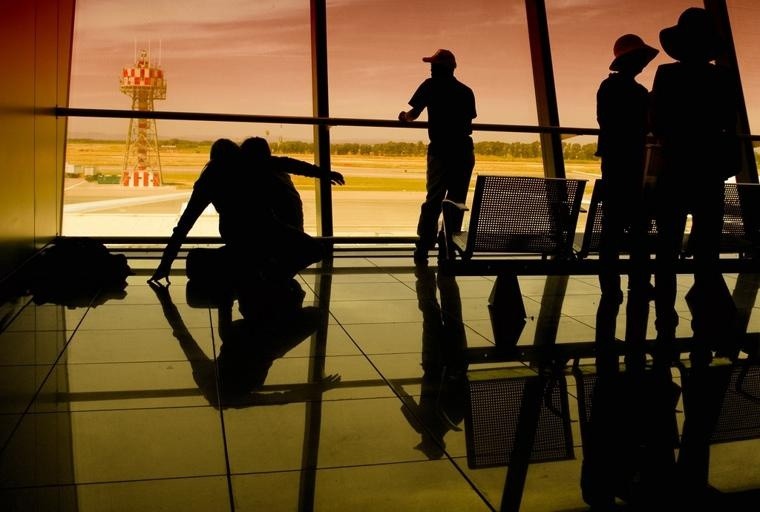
[
  {"xmin": 423, "ymin": 49, "xmax": 456, "ymax": 65},
  {"xmin": 610, "ymin": 8, "xmax": 729, "ymax": 70}
]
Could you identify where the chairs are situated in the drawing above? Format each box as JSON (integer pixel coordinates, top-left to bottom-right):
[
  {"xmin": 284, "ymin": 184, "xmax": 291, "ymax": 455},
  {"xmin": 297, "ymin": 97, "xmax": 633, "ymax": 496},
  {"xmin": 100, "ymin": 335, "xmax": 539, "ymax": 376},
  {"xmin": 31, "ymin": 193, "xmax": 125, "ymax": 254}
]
[
  {"xmin": 678, "ymin": 354, "xmax": 759, "ymax": 454},
  {"xmin": 573, "ymin": 363, "xmax": 685, "ymax": 457},
  {"xmin": 573, "ymin": 173, "xmax": 690, "ymax": 285},
  {"xmin": 456, "ymin": 361, "xmax": 579, "ymax": 469},
  {"xmin": 440, "ymin": 168, "xmax": 587, "ymax": 303},
  {"xmin": 697, "ymin": 177, "xmax": 757, "ymax": 274}
]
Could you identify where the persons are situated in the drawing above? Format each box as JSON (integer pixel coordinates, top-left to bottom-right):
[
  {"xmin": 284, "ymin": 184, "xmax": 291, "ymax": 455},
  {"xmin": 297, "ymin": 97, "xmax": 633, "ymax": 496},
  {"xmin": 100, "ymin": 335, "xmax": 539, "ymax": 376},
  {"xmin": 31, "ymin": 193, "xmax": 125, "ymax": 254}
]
[
  {"xmin": 595, "ymin": 34, "xmax": 659, "ymax": 301},
  {"xmin": 580, "ymin": 301, "xmax": 747, "ymax": 512},
  {"xmin": 240, "ymin": 136, "xmax": 345, "ymax": 233},
  {"xmin": 652, "ymin": 7, "xmax": 741, "ymax": 314},
  {"xmin": 398, "ymin": 49, "xmax": 476, "ymax": 257},
  {"xmin": 403, "ymin": 257, "xmax": 469, "ymax": 461},
  {"xmin": 147, "ymin": 139, "xmax": 325, "ymax": 282},
  {"xmin": 147, "ymin": 282, "xmax": 322, "ymax": 408}
]
